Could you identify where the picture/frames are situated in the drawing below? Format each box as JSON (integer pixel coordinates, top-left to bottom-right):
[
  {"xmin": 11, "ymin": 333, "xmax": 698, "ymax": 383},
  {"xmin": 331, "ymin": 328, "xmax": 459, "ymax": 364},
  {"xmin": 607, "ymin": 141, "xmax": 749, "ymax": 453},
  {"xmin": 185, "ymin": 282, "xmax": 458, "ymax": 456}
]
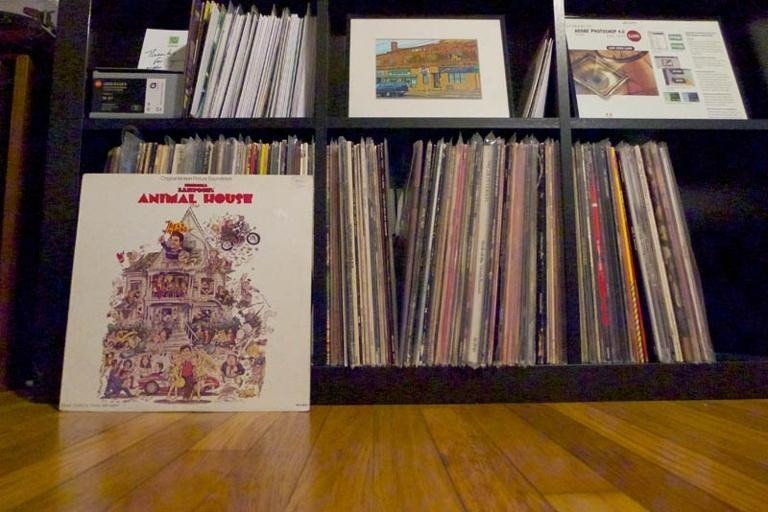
[
  {"xmin": 564, "ymin": 13, "xmax": 753, "ymax": 119},
  {"xmin": 346, "ymin": 12, "xmax": 516, "ymax": 120}
]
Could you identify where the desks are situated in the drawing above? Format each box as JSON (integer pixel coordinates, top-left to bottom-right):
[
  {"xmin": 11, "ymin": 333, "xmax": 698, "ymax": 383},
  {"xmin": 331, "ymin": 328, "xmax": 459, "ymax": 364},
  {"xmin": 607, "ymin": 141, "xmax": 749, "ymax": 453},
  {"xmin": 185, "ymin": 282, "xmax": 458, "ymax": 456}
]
[{"xmin": 1, "ymin": 10, "xmax": 69, "ymax": 392}]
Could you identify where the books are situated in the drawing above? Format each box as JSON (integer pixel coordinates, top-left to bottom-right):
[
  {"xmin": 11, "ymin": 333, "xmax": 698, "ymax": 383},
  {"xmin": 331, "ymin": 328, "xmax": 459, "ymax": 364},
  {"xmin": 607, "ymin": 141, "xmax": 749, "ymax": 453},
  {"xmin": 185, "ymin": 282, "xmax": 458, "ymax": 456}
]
[
  {"xmin": 518, "ymin": 33, "xmax": 554, "ymax": 120},
  {"xmin": 324, "ymin": 134, "xmax": 716, "ymax": 368},
  {"xmin": 102, "ymin": 128, "xmax": 315, "ymax": 175},
  {"xmin": 186, "ymin": 0, "xmax": 317, "ymax": 117}
]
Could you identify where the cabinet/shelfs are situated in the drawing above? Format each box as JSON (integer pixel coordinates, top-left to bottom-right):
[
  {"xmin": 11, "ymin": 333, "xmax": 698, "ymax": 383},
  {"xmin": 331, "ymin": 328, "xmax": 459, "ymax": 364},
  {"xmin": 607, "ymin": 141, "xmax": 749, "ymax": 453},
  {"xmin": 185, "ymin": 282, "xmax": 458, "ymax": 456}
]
[{"xmin": 33, "ymin": 0, "xmax": 768, "ymax": 407}]
